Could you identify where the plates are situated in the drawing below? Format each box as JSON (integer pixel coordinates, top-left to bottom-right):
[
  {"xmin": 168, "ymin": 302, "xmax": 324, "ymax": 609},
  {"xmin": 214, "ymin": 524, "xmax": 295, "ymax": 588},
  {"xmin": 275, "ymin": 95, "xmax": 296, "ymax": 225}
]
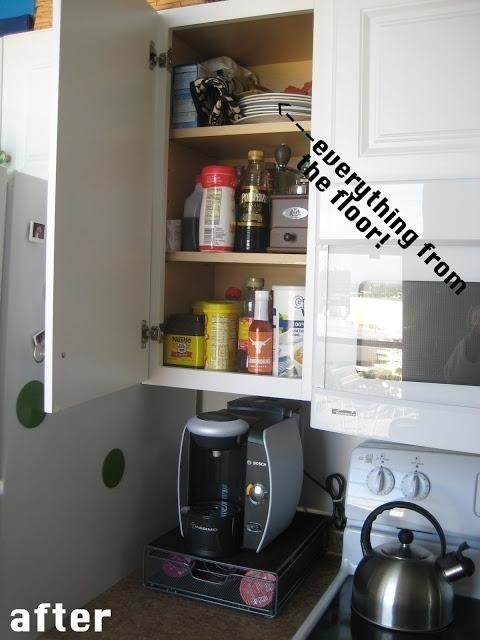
[{"xmin": 236, "ymin": 92, "xmax": 311, "ymax": 122}]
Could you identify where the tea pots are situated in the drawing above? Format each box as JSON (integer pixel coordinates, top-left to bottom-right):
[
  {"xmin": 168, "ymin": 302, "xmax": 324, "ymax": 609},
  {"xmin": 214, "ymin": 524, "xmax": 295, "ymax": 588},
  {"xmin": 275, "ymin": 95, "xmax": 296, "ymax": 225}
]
[{"xmin": 351, "ymin": 501, "xmax": 476, "ymax": 636}]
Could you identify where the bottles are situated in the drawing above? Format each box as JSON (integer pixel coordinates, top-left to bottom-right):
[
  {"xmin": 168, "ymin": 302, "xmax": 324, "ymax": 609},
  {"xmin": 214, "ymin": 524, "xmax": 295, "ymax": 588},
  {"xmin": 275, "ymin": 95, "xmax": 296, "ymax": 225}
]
[
  {"xmin": 224, "ymin": 270, "xmax": 275, "ymax": 374},
  {"xmin": 168, "ymin": 142, "xmax": 270, "ymax": 252}
]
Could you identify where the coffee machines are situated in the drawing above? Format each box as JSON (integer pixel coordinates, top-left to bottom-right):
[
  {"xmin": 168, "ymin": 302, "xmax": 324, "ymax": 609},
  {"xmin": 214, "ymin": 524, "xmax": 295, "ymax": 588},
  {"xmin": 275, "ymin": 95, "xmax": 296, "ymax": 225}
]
[{"xmin": 141, "ymin": 396, "xmax": 330, "ymax": 621}]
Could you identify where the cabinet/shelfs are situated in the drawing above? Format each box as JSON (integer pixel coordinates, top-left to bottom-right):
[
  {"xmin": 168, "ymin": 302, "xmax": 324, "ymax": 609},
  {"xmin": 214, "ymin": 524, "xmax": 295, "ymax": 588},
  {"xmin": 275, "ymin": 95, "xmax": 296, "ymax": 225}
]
[{"xmin": 45, "ymin": 0, "xmax": 480, "ymax": 455}]
